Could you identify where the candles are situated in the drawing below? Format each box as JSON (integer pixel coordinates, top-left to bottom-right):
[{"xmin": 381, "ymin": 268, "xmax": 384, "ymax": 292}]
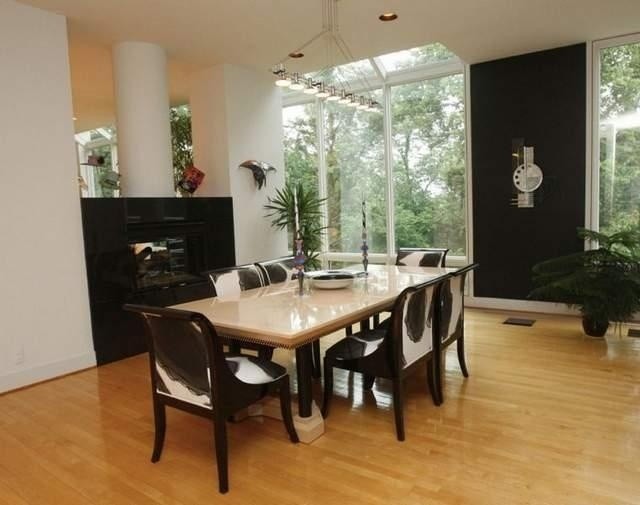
[
  {"xmin": 362, "ymin": 232, "xmax": 367, "ymax": 240},
  {"xmin": 295, "ymin": 239, "xmax": 304, "ymax": 249}
]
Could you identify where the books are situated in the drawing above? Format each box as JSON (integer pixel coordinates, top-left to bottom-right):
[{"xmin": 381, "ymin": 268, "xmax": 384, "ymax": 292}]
[{"xmin": 177, "ymin": 166, "xmax": 206, "ymax": 193}]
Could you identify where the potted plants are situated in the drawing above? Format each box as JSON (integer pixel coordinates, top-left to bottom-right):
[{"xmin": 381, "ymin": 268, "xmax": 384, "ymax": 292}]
[{"xmin": 525, "ymin": 224, "xmax": 640, "ymax": 337}]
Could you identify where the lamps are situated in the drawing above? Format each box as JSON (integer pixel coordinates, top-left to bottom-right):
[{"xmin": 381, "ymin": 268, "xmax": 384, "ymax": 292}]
[{"xmin": 266, "ymin": 1, "xmax": 385, "ymax": 113}]
